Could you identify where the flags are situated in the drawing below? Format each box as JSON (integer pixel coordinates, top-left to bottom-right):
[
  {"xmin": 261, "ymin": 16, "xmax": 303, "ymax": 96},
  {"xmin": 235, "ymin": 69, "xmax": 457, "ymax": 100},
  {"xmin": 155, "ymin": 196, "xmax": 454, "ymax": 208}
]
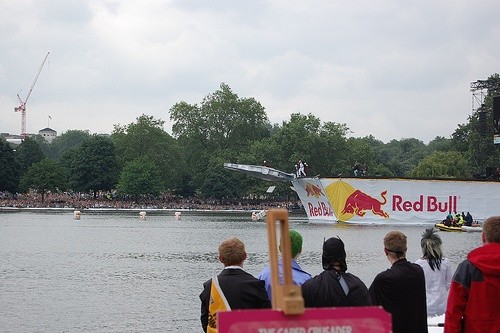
[{"xmin": 207, "ymin": 274, "xmax": 231, "ymax": 333}]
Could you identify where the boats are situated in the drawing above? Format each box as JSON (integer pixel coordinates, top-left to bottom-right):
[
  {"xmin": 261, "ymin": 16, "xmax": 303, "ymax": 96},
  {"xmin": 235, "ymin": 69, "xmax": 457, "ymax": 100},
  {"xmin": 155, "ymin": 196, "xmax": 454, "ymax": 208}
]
[
  {"xmin": 460, "ymin": 225, "xmax": 483, "ymax": 232},
  {"xmin": 433, "ymin": 223, "xmax": 481, "ymax": 232}
]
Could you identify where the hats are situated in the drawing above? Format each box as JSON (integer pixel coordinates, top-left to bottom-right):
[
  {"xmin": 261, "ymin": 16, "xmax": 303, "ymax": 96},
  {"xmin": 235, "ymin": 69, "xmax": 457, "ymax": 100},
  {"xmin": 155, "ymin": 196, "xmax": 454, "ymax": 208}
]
[{"xmin": 321, "ymin": 235, "xmax": 347, "ymax": 274}]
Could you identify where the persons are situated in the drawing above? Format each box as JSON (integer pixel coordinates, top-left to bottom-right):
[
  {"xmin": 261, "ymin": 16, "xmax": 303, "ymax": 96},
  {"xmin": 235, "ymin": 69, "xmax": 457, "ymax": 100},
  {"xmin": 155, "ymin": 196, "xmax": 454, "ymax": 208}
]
[
  {"xmin": 443, "ymin": 216, "xmax": 500, "ymax": 333},
  {"xmin": 448, "ymin": 212, "xmax": 453, "ymax": 218},
  {"xmin": 360, "ymin": 163, "xmax": 368, "ymax": 176},
  {"xmin": 257, "ymin": 230, "xmax": 311, "ymax": 302},
  {"xmin": 464, "ymin": 212, "xmax": 473, "ymax": 226},
  {"xmin": 414, "ymin": 229, "xmax": 452, "ymax": 333},
  {"xmin": 368, "ymin": 230, "xmax": 428, "ymax": 333},
  {"xmin": 443, "ymin": 216, "xmax": 451, "ymax": 227},
  {"xmin": 454, "ymin": 212, "xmax": 463, "ymax": 227},
  {"xmin": 353, "ymin": 163, "xmax": 358, "ymax": 176},
  {"xmin": 0, "ymin": 190, "xmax": 303, "ymax": 211},
  {"xmin": 296, "ymin": 159, "xmax": 308, "ymax": 177},
  {"xmin": 199, "ymin": 238, "xmax": 272, "ymax": 333},
  {"xmin": 461, "ymin": 212, "xmax": 465, "ymax": 220},
  {"xmin": 301, "ymin": 238, "xmax": 371, "ymax": 308}
]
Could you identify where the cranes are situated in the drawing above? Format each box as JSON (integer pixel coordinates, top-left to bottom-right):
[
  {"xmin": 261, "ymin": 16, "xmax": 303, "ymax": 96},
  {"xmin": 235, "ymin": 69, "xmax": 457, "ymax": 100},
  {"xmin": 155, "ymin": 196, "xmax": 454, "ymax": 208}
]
[{"xmin": 13, "ymin": 51, "xmax": 51, "ymax": 142}]
